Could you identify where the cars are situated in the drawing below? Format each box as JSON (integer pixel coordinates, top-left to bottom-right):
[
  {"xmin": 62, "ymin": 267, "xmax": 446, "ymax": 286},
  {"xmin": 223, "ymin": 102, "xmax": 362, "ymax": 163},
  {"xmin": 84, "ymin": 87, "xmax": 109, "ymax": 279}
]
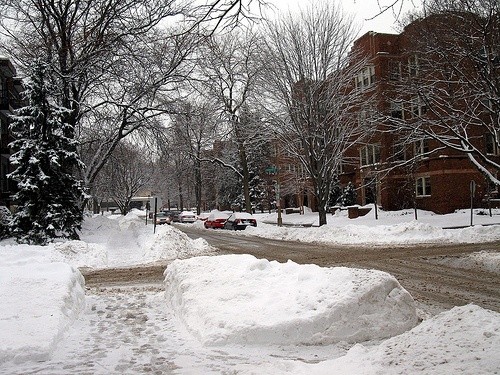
[
  {"xmin": 204, "ymin": 213, "xmax": 229, "ymax": 229},
  {"xmin": 224, "ymin": 213, "xmax": 257, "ymax": 231},
  {"xmin": 149, "ymin": 210, "xmax": 198, "ymax": 225}
]
